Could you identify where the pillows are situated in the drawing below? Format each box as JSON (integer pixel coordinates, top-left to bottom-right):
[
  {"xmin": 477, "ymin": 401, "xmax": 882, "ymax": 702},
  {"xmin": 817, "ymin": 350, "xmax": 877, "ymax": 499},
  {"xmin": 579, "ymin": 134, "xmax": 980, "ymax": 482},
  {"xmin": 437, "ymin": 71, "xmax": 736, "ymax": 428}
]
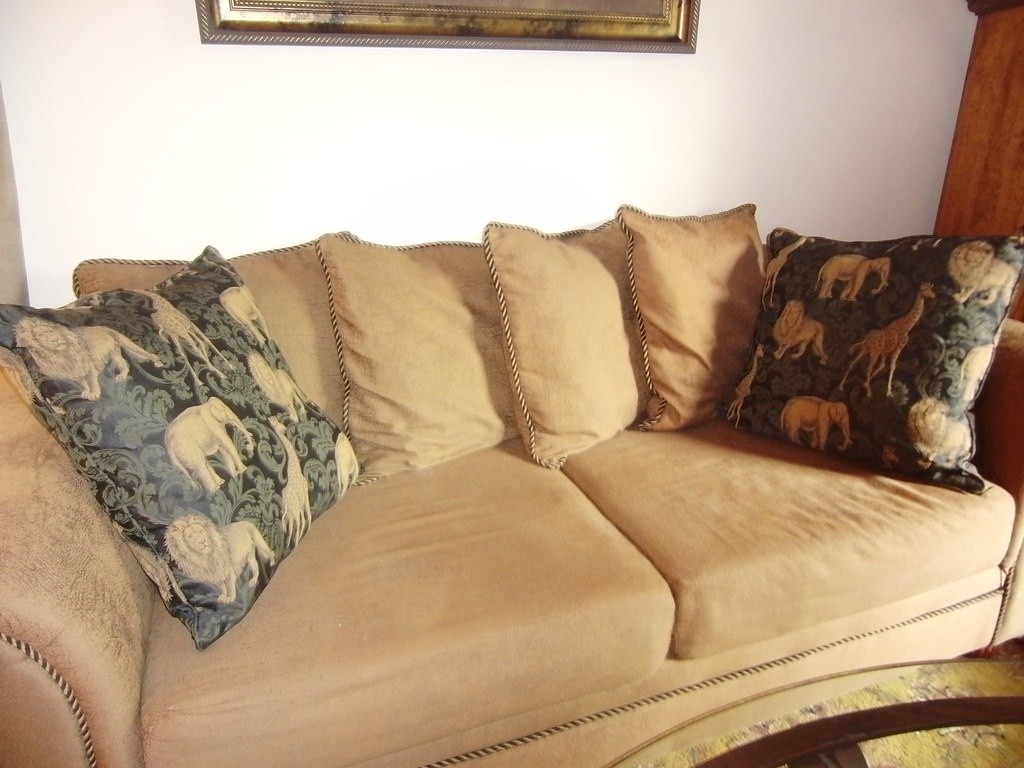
[
  {"xmin": 716, "ymin": 227, "xmax": 1024, "ymax": 495},
  {"xmin": 482, "ymin": 219, "xmax": 653, "ymax": 470},
  {"xmin": 72, "ymin": 230, "xmax": 358, "ymax": 435},
  {"xmin": 317, "ymin": 235, "xmax": 523, "ymax": 489},
  {"xmin": 613, "ymin": 202, "xmax": 770, "ymax": 434},
  {"xmin": 0, "ymin": 244, "xmax": 362, "ymax": 651}
]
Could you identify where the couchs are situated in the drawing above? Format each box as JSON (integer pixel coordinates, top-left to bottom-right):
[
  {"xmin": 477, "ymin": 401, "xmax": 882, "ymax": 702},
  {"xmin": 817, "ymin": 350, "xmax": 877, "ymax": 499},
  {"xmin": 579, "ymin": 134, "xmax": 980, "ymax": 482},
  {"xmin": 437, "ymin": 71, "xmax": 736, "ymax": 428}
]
[{"xmin": 0, "ymin": 323, "xmax": 1024, "ymax": 768}]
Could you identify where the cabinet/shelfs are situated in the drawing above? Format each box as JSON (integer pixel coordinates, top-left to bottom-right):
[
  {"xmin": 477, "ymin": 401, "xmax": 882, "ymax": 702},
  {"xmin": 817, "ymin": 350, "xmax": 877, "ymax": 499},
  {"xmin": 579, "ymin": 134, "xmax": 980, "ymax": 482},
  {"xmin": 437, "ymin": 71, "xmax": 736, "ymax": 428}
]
[{"xmin": 920, "ymin": 2, "xmax": 1023, "ymax": 323}]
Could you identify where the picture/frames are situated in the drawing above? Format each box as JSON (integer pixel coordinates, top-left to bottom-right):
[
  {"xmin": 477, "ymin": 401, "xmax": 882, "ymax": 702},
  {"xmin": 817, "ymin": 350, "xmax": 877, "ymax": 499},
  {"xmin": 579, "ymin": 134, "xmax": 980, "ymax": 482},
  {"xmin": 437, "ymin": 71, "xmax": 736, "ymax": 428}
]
[{"xmin": 193, "ymin": 0, "xmax": 701, "ymax": 55}]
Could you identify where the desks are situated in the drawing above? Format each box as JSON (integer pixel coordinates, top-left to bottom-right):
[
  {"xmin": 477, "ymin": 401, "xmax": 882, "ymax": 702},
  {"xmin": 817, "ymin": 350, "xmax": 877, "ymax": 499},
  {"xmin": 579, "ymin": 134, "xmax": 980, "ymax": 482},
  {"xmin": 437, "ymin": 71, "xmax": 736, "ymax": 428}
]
[{"xmin": 594, "ymin": 658, "xmax": 1023, "ymax": 767}]
